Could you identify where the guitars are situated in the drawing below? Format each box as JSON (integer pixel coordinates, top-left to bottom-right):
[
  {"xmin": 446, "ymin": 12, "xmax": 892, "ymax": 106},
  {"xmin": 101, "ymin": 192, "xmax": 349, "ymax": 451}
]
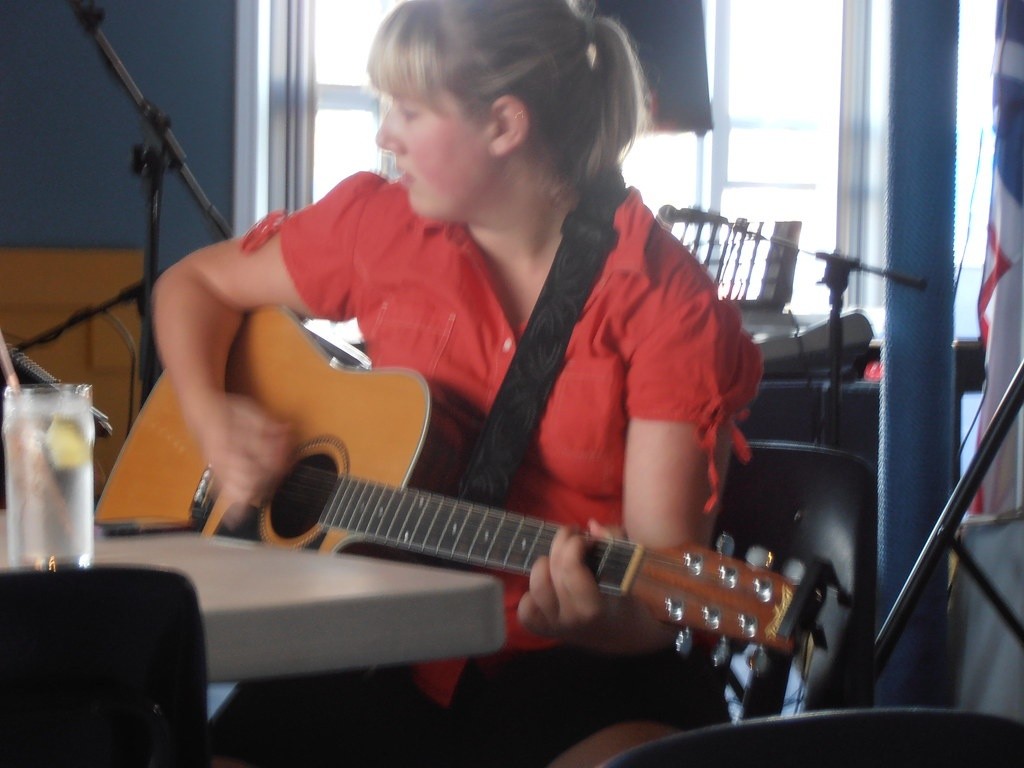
[{"xmin": 94, "ymin": 300, "xmax": 826, "ymax": 671}]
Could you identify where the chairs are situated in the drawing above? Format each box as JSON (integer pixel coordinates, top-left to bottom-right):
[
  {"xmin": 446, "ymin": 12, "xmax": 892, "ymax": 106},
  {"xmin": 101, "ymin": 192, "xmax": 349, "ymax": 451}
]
[{"xmin": 713, "ymin": 439, "xmax": 873, "ymax": 720}]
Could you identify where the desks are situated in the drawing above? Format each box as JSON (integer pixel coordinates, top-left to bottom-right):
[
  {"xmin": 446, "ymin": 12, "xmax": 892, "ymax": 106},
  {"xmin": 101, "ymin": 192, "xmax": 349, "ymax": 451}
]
[{"xmin": 0, "ymin": 507, "xmax": 503, "ymax": 685}]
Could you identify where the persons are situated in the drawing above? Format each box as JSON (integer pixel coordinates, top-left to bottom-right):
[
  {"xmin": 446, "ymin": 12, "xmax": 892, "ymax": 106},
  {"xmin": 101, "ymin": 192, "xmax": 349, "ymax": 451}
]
[{"xmin": 151, "ymin": 0, "xmax": 764, "ymax": 768}]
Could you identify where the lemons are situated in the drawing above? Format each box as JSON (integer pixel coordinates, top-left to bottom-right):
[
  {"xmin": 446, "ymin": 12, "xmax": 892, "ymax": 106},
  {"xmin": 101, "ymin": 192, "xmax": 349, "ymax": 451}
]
[{"xmin": 45, "ymin": 414, "xmax": 89, "ymax": 469}]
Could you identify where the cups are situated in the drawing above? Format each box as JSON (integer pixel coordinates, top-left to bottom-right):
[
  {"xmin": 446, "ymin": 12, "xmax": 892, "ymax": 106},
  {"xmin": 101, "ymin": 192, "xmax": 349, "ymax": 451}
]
[{"xmin": 3, "ymin": 382, "xmax": 94, "ymax": 569}]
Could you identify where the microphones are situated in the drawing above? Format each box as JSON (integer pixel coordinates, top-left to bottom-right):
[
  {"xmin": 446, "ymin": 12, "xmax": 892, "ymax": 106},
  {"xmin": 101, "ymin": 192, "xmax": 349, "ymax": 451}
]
[{"xmin": 656, "ymin": 206, "xmax": 728, "ymax": 225}]
